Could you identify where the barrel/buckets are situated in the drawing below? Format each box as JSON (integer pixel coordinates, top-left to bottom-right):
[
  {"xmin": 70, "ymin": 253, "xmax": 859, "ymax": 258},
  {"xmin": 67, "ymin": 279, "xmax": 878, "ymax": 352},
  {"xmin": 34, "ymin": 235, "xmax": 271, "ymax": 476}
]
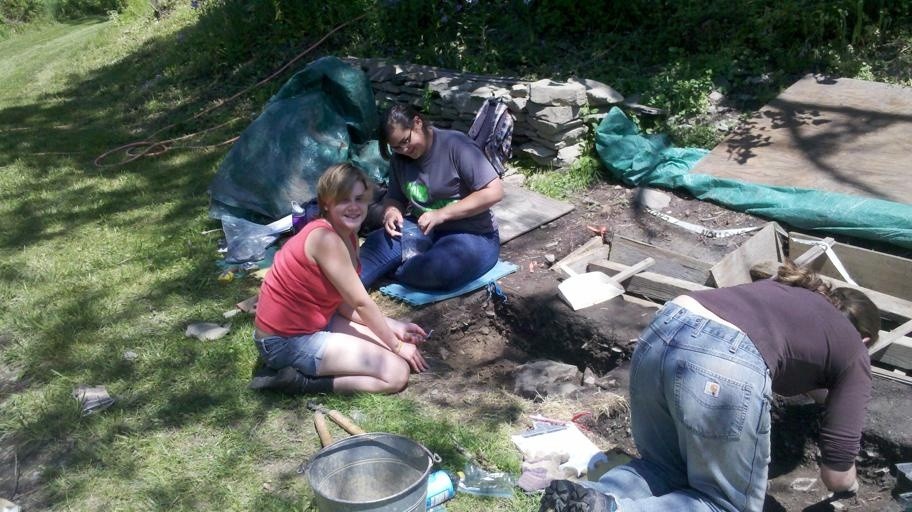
[{"xmin": 296, "ymin": 432, "xmax": 442, "ymax": 512}]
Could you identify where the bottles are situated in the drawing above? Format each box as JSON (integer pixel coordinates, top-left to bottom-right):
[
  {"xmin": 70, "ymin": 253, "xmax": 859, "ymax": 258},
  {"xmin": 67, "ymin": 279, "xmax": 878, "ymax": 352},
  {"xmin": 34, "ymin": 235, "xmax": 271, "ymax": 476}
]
[
  {"xmin": 289, "ymin": 200, "xmax": 319, "ymax": 233},
  {"xmin": 425, "ymin": 469, "xmax": 465, "ymax": 510}
]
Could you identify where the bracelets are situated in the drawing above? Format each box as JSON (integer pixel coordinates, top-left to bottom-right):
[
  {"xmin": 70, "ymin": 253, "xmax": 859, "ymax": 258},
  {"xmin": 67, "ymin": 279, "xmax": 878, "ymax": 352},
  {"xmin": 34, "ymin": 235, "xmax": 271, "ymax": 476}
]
[{"xmin": 394, "ymin": 339, "xmax": 404, "ymax": 355}]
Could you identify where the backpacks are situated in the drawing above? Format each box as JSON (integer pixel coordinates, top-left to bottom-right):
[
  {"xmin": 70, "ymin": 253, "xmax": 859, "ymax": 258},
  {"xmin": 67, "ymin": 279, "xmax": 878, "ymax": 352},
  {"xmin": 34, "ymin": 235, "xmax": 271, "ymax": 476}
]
[
  {"xmin": 358, "ymin": 180, "xmax": 388, "ymax": 237},
  {"xmin": 293, "ymin": 200, "xmax": 321, "ymax": 233},
  {"xmin": 468, "ymin": 96, "xmax": 514, "ymax": 175}
]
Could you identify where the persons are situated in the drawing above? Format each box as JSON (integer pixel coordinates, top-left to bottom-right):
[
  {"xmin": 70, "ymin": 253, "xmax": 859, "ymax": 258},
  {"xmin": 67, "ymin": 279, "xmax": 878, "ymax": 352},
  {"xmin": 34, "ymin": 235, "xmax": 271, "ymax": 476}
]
[
  {"xmin": 539, "ymin": 257, "xmax": 882, "ymax": 512},
  {"xmin": 249, "ymin": 160, "xmax": 431, "ymax": 393},
  {"xmin": 351, "ymin": 101, "xmax": 507, "ymax": 290}
]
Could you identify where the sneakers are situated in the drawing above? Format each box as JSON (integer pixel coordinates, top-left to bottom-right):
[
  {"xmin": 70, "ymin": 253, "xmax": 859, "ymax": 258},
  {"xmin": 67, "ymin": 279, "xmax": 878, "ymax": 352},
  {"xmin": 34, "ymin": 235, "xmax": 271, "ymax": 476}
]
[
  {"xmin": 538, "ymin": 480, "xmax": 611, "ymax": 512},
  {"xmin": 250, "ymin": 367, "xmax": 308, "ymax": 395}
]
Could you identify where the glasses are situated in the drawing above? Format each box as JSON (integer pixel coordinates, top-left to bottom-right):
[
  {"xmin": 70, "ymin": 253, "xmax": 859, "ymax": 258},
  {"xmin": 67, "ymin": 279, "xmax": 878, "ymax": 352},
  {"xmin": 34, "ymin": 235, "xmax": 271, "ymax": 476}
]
[{"xmin": 390, "ymin": 129, "xmax": 411, "ymax": 152}]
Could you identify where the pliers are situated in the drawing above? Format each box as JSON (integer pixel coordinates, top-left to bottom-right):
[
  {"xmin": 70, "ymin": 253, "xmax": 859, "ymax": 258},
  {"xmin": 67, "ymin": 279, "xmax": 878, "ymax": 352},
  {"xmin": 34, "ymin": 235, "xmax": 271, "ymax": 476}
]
[{"xmin": 307, "ymin": 399, "xmax": 367, "ymax": 448}]
